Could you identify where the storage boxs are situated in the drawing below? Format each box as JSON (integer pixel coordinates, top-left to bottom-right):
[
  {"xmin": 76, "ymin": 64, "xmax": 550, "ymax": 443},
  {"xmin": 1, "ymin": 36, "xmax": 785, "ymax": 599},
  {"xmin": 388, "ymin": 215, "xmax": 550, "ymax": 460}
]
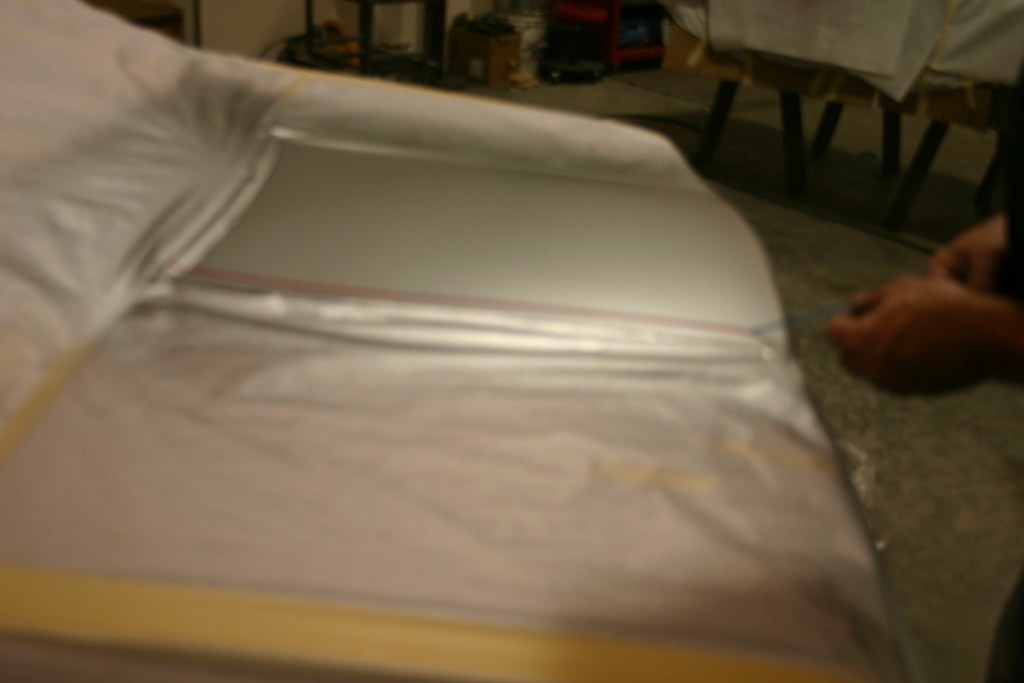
[{"xmin": 449, "ymin": 25, "xmax": 521, "ymax": 90}]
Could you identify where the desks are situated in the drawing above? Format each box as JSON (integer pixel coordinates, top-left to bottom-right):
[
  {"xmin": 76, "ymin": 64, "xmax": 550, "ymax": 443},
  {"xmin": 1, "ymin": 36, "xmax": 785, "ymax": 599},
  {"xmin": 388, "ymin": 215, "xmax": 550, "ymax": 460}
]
[{"xmin": 665, "ymin": 21, "xmax": 990, "ymax": 231}]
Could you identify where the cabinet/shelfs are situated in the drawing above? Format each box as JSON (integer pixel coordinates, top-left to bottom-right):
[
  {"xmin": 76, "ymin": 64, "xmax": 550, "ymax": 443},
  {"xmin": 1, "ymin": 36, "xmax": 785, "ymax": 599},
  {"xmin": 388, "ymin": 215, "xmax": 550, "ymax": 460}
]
[{"xmin": 550, "ymin": 0, "xmax": 666, "ymax": 74}]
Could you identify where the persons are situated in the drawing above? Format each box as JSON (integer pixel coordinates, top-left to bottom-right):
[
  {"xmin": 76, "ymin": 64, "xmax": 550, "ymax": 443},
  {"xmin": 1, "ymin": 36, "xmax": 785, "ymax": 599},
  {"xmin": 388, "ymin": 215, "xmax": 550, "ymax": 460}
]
[{"xmin": 827, "ymin": 47, "xmax": 1024, "ymax": 682}]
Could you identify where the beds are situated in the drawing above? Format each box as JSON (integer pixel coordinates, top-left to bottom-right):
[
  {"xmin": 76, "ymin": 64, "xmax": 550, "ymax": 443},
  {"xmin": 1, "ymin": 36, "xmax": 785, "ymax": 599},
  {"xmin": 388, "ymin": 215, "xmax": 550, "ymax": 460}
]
[{"xmin": 0, "ymin": 0, "xmax": 916, "ymax": 683}]
